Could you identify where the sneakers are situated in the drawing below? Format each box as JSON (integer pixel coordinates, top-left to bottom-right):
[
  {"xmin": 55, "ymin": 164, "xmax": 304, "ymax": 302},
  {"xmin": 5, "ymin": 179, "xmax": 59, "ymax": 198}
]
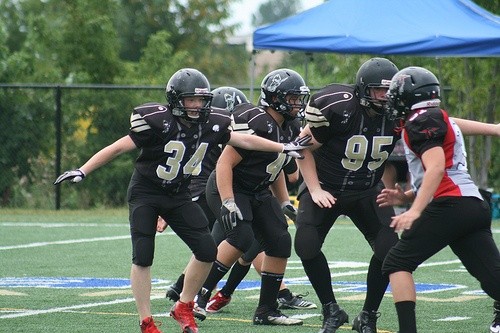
[
  {"xmin": 351, "ymin": 309, "xmax": 381, "ymax": 333},
  {"xmin": 138, "ymin": 315, "xmax": 162, "ymax": 333},
  {"xmin": 205, "ymin": 291, "xmax": 232, "ymax": 312},
  {"xmin": 276, "ymin": 291, "xmax": 318, "ymax": 309},
  {"xmin": 191, "ymin": 287, "xmax": 212, "ymax": 321},
  {"xmin": 170, "ymin": 300, "xmax": 198, "ymax": 333},
  {"xmin": 489, "ymin": 301, "xmax": 500, "ymax": 333},
  {"xmin": 253, "ymin": 307, "xmax": 303, "ymax": 326},
  {"xmin": 166, "ymin": 283, "xmax": 182, "ymax": 302},
  {"xmin": 316, "ymin": 301, "xmax": 349, "ymax": 333}
]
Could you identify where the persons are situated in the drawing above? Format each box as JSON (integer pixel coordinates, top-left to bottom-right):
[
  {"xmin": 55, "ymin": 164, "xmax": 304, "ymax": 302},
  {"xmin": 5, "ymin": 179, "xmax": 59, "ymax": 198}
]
[
  {"xmin": 372, "ymin": 65, "xmax": 500, "ymax": 333},
  {"xmin": 159, "ymin": 68, "xmax": 328, "ymax": 326},
  {"xmin": 53, "ymin": 66, "xmax": 314, "ymax": 332},
  {"xmin": 288, "ymin": 57, "xmax": 500, "ymax": 333}
]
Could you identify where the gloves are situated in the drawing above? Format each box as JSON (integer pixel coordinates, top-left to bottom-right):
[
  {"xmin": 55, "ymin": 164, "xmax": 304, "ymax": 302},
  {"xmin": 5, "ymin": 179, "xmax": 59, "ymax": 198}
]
[
  {"xmin": 53, "ymin": 169, "xmax": 87, "ymax": 185},
  {"xmin": 221, "ymin": 199, "xmax": 243, "ymax": 232},
  {"xmin": 281, "ymin": 133, "xmax": 315, "ymax": 160},
  {"xmin": 280, "ymin": 200, "xmax": 298, "ymax": 230}
]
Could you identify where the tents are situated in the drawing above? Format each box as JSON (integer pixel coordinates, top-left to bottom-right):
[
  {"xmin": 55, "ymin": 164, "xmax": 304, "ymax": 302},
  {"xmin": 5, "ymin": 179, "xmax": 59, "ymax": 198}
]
[{"xmin": 251, "ymin": 0, "xmax": 500, "ymax": 115}]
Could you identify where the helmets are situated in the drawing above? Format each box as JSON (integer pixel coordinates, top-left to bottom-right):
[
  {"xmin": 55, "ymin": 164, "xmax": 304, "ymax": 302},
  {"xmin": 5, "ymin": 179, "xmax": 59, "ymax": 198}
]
[
  {"xmin": 383, "ymin": 67, "xmax": 442, "ymax": 124},
  {"xmin": 355, "ymin": 57, "xmax": 399, "ymax": 121},
  {"xmin": 260, "ymin": 69, "xmax": 311, "ymax": 120},
  {"xmin": 165, "ymin": 68, "xmax": 214, "ymax": 125},
  {"xmin": 210, "ymin": 86, "xmax": 250, "ymax": 111}
]
